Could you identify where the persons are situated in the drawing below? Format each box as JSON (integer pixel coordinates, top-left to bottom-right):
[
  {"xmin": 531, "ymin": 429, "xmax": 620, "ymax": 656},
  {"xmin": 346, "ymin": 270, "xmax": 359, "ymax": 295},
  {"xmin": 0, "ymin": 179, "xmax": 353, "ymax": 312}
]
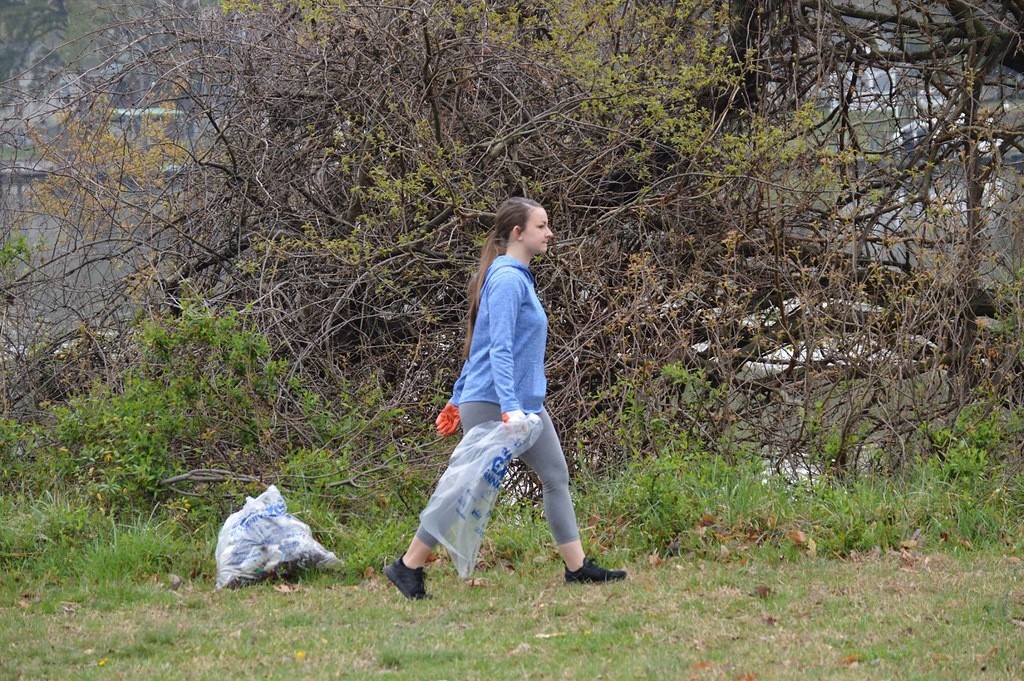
[{"xmin": 383, "ymin": 197, "xmax": 626, "ymax": 601}]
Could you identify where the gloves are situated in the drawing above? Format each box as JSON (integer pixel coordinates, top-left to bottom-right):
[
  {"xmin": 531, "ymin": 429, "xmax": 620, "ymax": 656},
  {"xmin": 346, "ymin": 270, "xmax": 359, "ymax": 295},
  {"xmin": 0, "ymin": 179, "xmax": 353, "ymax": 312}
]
[
  {"xmin": 503, "ymin": 410, "xmax": 527, "ymax": 436},
  {"xmin": 436, "ymin": 403, "xmax": 461, "ymax": 437}
]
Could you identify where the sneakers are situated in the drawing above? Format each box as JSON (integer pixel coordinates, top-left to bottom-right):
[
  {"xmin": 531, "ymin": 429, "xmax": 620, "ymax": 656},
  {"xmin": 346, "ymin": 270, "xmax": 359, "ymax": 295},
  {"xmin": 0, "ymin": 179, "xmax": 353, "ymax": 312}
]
[
  {"xmin": 383, "ymin": 551, "xmax": 433, "ymax": 602},
  {"xmin": 563, "ymin": 556, "xmax": 626, "ymax": 585}
]
